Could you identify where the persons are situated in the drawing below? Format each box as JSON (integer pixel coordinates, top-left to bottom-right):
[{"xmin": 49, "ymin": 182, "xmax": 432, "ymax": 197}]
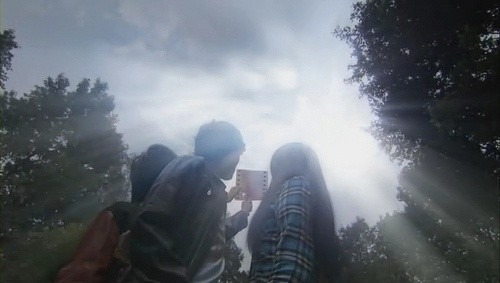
[
  {"xmin": 55, "ymin": 143, "xmax": 239, "ymax": 283},
  {"xmin": 116, "ymin": 119, "xmax": 253, "ymax": 283},
  {"xmin": 247, "ymin": 142, "xmax": 338, "ymax": 283}
]
